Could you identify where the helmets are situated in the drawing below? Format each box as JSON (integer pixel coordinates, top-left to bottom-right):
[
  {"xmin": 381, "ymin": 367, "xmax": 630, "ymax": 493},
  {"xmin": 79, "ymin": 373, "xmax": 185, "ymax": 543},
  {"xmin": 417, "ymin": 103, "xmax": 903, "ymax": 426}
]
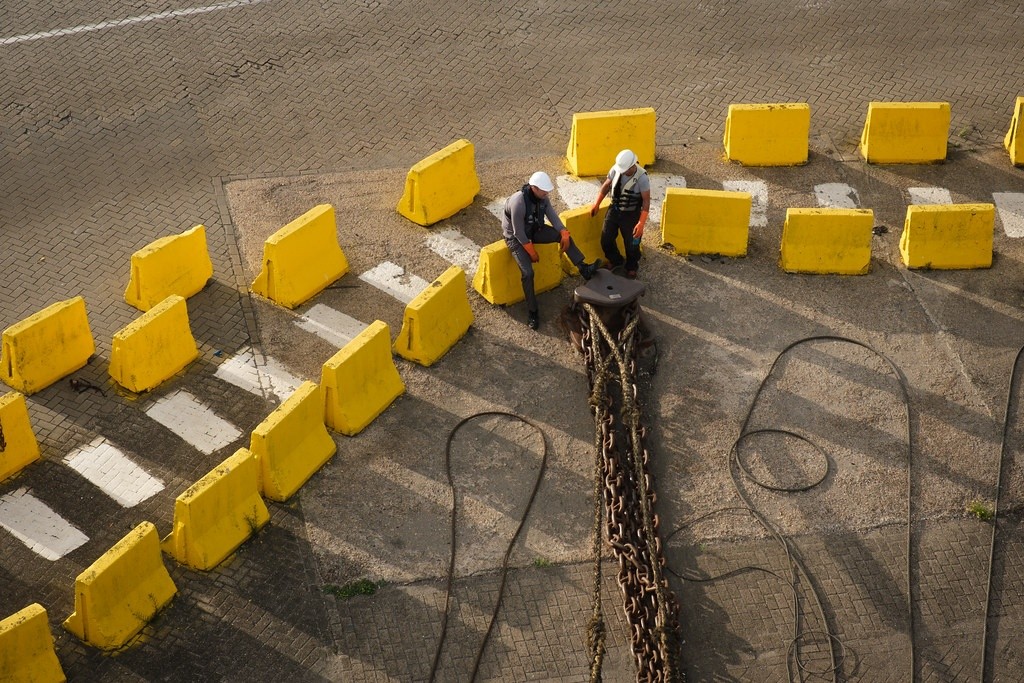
[
  {"xmin": 613, "ymin": 149, "xmax": 637, "ymax": 174},
  {"xmin": 529, "ymin": 171, "xmax": 554, "ymax": 192}
]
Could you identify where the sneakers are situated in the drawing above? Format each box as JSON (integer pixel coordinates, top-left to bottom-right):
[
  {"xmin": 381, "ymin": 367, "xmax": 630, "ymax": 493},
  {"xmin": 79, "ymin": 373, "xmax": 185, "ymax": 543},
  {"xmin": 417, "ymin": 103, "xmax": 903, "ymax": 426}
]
[
  {"xmin": 579, "ymin": 258, "xmax": 602, "ymax": 280},
  {"xmin": 528, "ymin": 309, "xmax": 539, "ymax": 330}
]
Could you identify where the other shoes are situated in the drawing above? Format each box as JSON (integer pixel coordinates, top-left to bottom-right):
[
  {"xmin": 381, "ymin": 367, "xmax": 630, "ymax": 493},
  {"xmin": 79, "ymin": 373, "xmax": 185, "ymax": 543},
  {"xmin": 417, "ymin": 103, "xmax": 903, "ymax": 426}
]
[
  {"xmin": 626, "ymin": 269, "xmax": 636, "ymax": 280},
  {"xmin": 601, "ymin": 262, "xmax": 623, "ymax": 271}
]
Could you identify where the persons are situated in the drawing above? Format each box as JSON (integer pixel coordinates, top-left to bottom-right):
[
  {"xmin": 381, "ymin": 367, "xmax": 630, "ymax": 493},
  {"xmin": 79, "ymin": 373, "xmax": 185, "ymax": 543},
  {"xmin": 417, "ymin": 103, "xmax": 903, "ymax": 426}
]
[
  {"xmin": 502, "ymin": 172, "xmax": 602, "ymax": 330},
  {"xmin": 590, "ymin": 149, "xmax": 650, "ymax": 276}
]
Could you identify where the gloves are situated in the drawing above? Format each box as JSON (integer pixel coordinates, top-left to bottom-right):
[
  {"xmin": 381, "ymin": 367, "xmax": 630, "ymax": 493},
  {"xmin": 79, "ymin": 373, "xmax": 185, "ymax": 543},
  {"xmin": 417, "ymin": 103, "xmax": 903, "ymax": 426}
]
[
  {"xmin": 632, "ymin": 210, "xmax": 648, "ymax": 239},
  {"xmin": 590, "ymin": 192, "xmax": 606, "ymax": 217},
  {"xmin": 560, "ymin": 228, "xmax": 571, "ymax": 253},
  {"xmin": 523, "ymin": 239, "xmax": 539, "ymax": 263}
]
